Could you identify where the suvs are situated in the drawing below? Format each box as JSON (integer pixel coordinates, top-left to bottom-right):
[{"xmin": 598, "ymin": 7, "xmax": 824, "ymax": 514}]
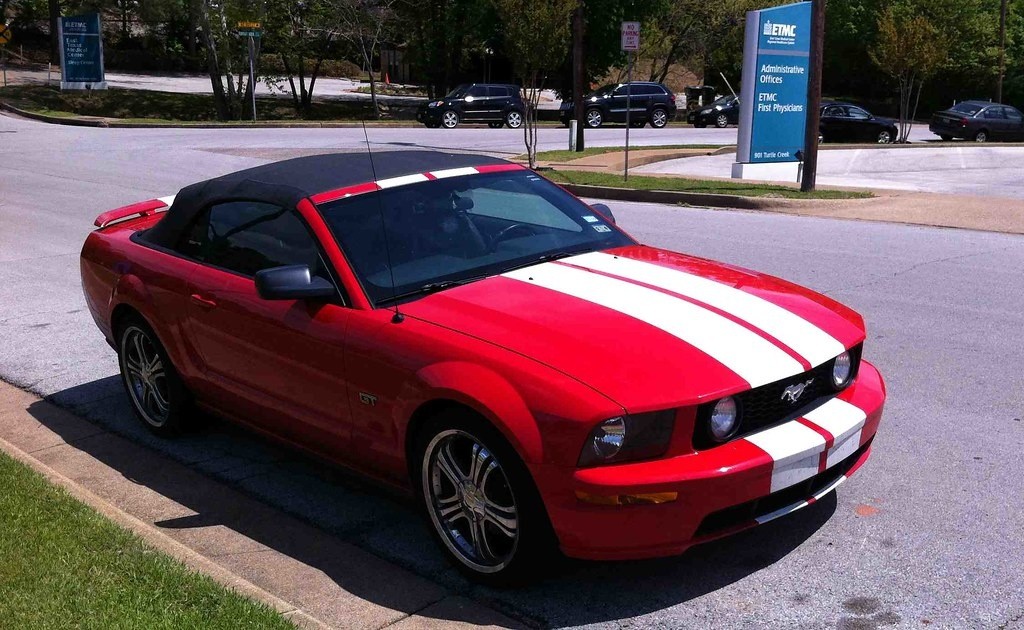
[
  {"xmin": 563, "ymin": 81, "xmax": 677, "ymax": 129},
  {"xmin": 414, "ymin": 84, "xmax": 525, "ymax": 130}
]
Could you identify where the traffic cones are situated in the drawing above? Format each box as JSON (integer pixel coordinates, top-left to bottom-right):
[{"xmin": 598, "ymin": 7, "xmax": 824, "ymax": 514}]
[{"xmin": 385, "ymin": 73, "xmax": 390, "ymax": 84}]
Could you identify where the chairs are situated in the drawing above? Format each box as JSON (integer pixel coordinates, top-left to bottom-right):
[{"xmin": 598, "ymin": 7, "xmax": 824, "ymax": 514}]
[{"xmin": 341, "ymin": 195, "xmax": 487, "ymax": 279}]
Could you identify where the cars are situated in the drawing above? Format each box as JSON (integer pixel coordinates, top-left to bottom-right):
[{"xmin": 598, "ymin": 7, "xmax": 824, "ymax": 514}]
[
  {"xmin": 930, "ymin": 100, "xmax": 1024, "ymax": 144},
  {"xmin": 79, "ymin": 146, "xmax": 886, "ymax": 581},
  {"xmin": 687, "ymin": 94, "xmax": 740, "ymax": 129},
  {"xmin": 819, "ymin": 102, "xmax": 897, "ymax": 145}
]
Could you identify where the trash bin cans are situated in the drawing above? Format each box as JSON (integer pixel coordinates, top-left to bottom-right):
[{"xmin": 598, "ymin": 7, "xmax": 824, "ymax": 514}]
[{"xmin": 684, "ymin": 86, "xmax": 716, "ymax": 125}]
